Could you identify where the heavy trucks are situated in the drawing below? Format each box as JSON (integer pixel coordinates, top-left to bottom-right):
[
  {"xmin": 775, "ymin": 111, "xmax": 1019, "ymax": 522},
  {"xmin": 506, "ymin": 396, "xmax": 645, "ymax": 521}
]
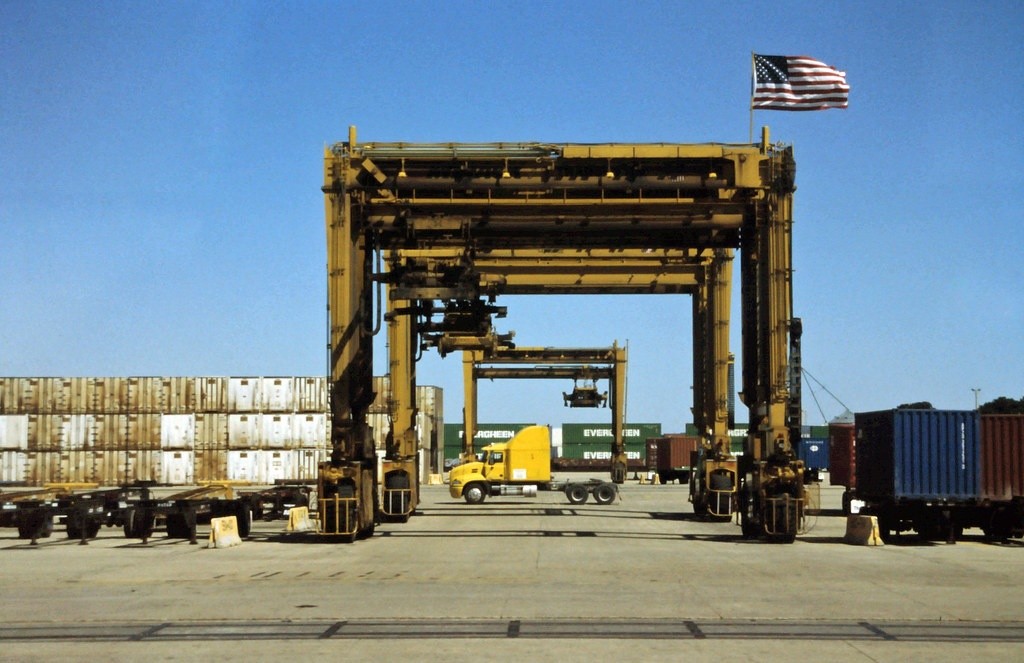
[{"xmin": 450, "ymin": 426, "xmax": 617, "ymax": 505}]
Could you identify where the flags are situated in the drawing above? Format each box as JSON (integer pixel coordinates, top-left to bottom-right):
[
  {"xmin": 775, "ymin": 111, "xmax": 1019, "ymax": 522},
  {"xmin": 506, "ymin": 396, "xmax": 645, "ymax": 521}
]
[{"xmin": 748, "ymin": 53, "xmax": 850, "ymax": 111}]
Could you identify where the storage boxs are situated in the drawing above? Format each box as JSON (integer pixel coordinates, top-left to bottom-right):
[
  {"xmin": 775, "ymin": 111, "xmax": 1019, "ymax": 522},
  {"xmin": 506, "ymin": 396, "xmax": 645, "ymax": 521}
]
[
  {"xmin": 445, "ymin": 410, "xmax": 1024, "ymax": 520},
  {"xmin": 0, "ymin": 376, "xmax": 444, "ymax": 500}
]
